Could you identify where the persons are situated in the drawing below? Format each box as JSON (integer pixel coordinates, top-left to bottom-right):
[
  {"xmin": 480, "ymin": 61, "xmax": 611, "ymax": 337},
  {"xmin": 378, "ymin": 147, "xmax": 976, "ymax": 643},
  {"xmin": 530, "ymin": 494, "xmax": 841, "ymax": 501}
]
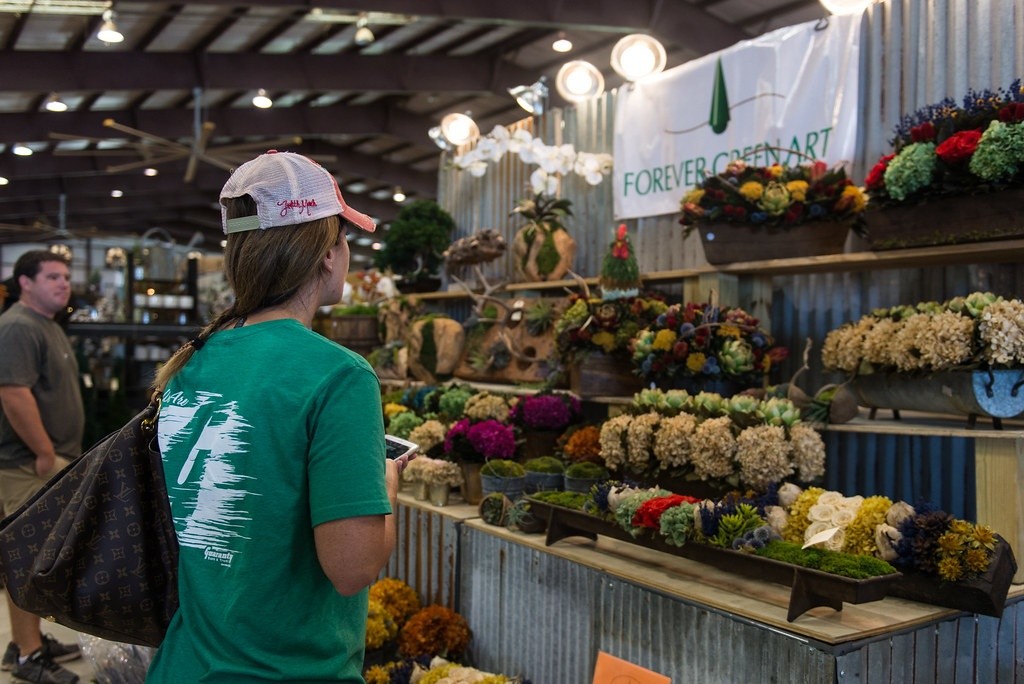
[
  {"xmin": 0, "ymin": 250, "xmax": 85, "ymax": 684},
  {"xmin": 145, "ymin": 149, "xmax": 416, "ymax": 684}
]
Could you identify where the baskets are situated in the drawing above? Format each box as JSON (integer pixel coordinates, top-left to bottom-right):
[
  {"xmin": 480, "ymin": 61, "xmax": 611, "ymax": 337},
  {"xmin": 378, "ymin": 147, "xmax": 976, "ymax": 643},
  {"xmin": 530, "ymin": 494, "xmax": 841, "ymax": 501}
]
[
  {"xmin": 864, "ymin": 182, "xmax": 1023, "ymax": 249},
  {"xmin": 578, "ymin": 356, "xmax": 643, "ymax": 397},
  {"xmin": 696, "ymin": 147, "xmax": 850, "ymax": 265}
]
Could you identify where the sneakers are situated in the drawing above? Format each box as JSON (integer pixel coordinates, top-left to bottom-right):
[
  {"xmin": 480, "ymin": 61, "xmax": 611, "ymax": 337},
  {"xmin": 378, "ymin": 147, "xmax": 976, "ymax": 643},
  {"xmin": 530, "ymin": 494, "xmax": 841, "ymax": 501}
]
[
  {"xmin": 1, "ymin": 632, "xmax": 81, "ymax": 672},
  {"xmin": 9, "ymin": 647, "xmax": 80, "ymax": 684}
]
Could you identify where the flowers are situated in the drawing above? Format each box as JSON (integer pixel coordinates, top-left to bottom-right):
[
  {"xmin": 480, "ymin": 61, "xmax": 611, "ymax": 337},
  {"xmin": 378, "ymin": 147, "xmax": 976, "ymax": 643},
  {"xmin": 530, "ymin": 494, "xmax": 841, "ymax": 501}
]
[
  {"xmin": 673, "ymin": 154, "xmax": 875, "ymax": 244},
  {"xmin": 363, "ymin": 574, "xmax": 525, "ymax": 684},
  {"xmin": 823, "ymin": 291, "xmax": 1024, "ymax": 374},
  {"xmin": 862, "ymin": 78, "xmax": 1023, "ymax": 207},
  {"xmin": 547, "ymin": 290, "xmax": 789, "ymax": 383},
  {"xmin": 388, "ymin": 384, "xmax": 581, "ymax": 463}
]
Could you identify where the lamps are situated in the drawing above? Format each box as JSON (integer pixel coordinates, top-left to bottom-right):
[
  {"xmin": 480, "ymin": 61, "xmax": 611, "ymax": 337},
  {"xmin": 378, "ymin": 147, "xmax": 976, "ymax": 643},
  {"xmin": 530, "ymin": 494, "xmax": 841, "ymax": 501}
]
[
  {"xmin": 504, "ymin": 73, "xmax": 550, "ymax": 118},
  {"xmin": 96, "ymin": 13, "xmax": 125, "ymax": 42},
  {"xmin": 555, "ymin": 57, "xmax": 605, "ymax": 103},
  {"xmin": 552, "ymin": 30, "xmax": 573, "ymax": 52},
  {"xmin": 608, "ymin": 31, "xmax": 667, "ymax": 82},
  {"xmin": 437, "ymin": 107, "xmax": 482, "ymax": 146},
  {"xmin": 355, "ymin": 18, "xmax": 376, "ymax": 46}
]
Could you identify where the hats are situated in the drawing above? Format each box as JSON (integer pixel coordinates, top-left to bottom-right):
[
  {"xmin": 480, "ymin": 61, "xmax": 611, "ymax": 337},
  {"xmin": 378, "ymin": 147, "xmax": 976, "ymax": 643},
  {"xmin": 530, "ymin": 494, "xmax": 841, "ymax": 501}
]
[{"xmin": 220, "ymin": 148, "xmax": 377, "ymax": 234}]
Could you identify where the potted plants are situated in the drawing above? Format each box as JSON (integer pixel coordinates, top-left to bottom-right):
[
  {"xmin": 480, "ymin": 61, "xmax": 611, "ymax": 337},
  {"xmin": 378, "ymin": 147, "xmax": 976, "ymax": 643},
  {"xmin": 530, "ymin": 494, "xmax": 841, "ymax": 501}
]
[
  {"xmin": 373, "ymin": 198, "xmax": 457, "ymax": 292},
  {"xmin": 478, "ymin": 384, "xmax": 1015, "ymax": 621}
]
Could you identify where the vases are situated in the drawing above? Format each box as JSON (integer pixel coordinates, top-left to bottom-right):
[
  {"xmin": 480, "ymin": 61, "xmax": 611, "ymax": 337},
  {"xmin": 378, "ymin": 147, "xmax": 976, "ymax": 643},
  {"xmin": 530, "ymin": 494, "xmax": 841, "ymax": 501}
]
[
  {"xmin": 841, "ymin": 367, "xmax": 1024, "ymax": 428},
  {"xmin": 864, "ymin": 178, "xmax": 1024, "ymax": 254}
]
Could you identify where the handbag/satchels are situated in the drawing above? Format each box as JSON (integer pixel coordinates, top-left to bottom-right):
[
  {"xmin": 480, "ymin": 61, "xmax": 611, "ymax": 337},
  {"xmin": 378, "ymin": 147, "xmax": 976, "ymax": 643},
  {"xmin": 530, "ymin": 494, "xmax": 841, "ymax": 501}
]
[{"xmin": 0, "ymin": 398, "xmax": 178, "ymax": 649}]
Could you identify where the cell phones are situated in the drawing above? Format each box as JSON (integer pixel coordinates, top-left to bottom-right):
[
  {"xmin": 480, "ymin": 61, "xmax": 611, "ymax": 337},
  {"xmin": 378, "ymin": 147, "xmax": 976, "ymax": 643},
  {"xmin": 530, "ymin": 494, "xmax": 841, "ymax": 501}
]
[{"xmin": 383, "ymin": 434, "xmax": 419, "ymax": 461}]
